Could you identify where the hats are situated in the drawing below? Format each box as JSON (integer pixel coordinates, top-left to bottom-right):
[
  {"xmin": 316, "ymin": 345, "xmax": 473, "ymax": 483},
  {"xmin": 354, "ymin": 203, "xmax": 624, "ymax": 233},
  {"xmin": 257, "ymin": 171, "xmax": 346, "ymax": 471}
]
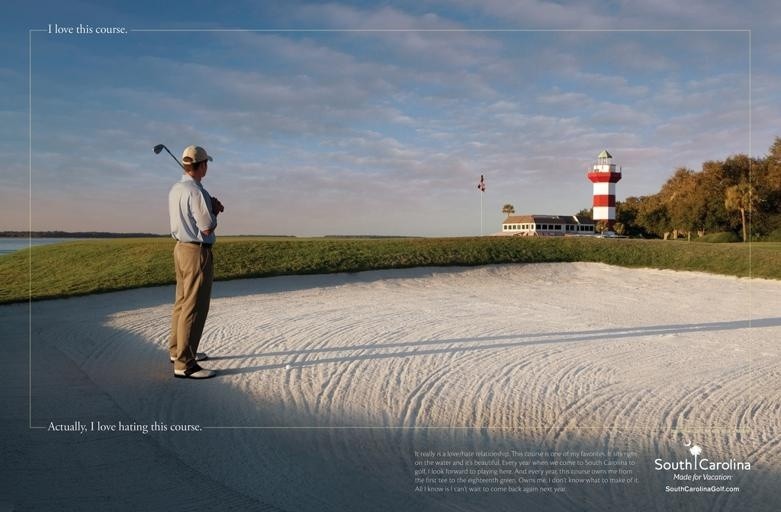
[{"xmin": 182, "ymin": 145, "xmax": 213, "ymax": 165}]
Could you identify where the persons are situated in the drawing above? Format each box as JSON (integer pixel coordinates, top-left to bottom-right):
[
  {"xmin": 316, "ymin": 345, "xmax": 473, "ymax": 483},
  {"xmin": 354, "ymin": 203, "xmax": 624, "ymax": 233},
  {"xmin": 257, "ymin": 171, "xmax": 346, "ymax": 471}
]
[{"xmin": 168, "ymin": 145, "xmax": 225, "ymax": 378}]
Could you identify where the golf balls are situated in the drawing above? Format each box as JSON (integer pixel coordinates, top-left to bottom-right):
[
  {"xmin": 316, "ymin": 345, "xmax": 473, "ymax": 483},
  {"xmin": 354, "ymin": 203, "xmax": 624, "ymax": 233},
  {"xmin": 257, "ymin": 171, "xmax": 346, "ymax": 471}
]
[{"xmin": 285, "ymin": 364, "xmax": 292, "ymax": 370}]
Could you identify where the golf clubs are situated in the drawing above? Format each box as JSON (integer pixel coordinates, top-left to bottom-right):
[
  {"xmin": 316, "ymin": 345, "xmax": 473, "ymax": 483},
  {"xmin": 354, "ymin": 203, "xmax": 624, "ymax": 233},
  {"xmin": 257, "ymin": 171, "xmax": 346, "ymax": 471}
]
[{"xmin": 152, "ymin": 144, "xmax": 225, "ymax": 213}]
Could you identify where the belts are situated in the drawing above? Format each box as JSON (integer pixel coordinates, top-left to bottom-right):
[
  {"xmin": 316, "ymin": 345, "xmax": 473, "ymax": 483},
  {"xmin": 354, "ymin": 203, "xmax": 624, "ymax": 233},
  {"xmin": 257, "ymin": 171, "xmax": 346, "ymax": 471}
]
[{"xmin": 190, "ymin": 241, "xmax": 213, "ymax": 248}]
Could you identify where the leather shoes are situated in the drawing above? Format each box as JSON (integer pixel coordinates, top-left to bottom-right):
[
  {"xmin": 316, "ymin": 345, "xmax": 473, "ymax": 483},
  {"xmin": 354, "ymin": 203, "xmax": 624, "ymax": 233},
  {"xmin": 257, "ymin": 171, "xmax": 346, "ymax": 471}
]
[
  {"xmin": 174, "ymin": 366, "xmax": 216, "ymax": 379},
  {"xmin": 170, "ymin": 351, "xmax": 207, "ymax": 362}
]
